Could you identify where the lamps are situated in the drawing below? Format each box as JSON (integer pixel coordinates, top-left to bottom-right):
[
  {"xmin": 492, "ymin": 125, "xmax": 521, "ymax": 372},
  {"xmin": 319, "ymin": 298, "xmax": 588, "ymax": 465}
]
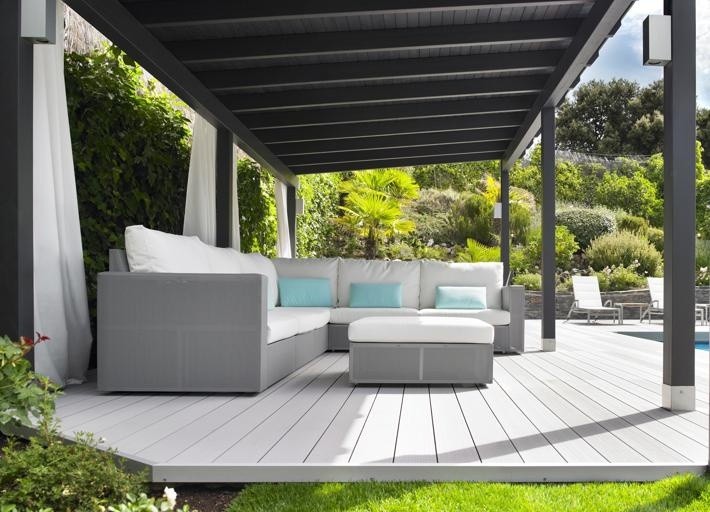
[
  {"xmin": 643, "ymin": 15, "xmax": 672, "ymax": 66},
  {"xmin": 296, "ymin": 199, "xmax": 304, "ymax": 216},
  {"xmin": 21, "ymin": 0, "xmax": 56, "ymax": 45},
  {"xmin": 494, "ymin": 203, "xmax": 502, "ymax": 218}
]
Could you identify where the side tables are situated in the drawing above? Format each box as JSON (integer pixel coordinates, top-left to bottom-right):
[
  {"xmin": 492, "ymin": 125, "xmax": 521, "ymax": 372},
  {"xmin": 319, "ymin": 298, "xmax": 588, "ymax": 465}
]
[
  {"xmin": 695, "ymin": 304, "xmax": 710, "ymax": 326},
  {"xmin": 613, "ymin": 303, "xmax": 651, "ymax": 324}
]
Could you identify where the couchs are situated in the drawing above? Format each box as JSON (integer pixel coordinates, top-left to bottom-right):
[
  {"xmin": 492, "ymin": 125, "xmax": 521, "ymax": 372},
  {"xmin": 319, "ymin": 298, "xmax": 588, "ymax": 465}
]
[{"xmin": 98, "ymin": 249, "xmax": 525, "ymax": 396}]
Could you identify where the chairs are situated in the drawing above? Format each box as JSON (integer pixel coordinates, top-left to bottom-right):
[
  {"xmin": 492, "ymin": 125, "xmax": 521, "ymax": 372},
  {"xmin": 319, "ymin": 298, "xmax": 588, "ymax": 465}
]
[
  {"xmin": 563, "ymin": 275, "xmax": 620, "ymax": 326},
  {"xmin": 640, "ymin": 277, "xmax": 704, "ymax": 326}
]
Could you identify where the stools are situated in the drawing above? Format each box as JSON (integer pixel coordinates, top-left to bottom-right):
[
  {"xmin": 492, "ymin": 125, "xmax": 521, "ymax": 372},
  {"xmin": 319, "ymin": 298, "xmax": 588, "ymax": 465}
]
[{"xmin": 348, "ymin": 315, "xmax": 495, "ymax": 386}]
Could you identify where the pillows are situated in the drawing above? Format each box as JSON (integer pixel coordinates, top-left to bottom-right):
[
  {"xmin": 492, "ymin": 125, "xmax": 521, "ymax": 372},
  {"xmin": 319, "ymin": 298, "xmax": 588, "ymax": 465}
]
[
  {"xmin": 241, "ymin": 252, "xmax": 281, "ymax": 305},
  {"xmin": 278, "ymin": 277, "xmax": 333, "ymax": 307},
  {"xmin": 271, "ymin": 257, "xmax": 339, "ymax": 307},
  {"xmin": 419, "ymin": 258, "xmax": 504, "ymax": 309},
  {"xmin": 434, "ymin": 286, "xmax": 487, "ymax": 309},
  {"xmin": 202, "ymin": 241, "xmax": 274, "ymax": 310},
  {"xmin": 348, "ymin": 282, "xmax": 402, "ymax": 307},
  {"xmin": 124, "ymin": 224, "xmax": 214, "ymax": 273},
  {"xmin": 334, "ymin": 255, "xmax": 421, "ymax": 310}
]
